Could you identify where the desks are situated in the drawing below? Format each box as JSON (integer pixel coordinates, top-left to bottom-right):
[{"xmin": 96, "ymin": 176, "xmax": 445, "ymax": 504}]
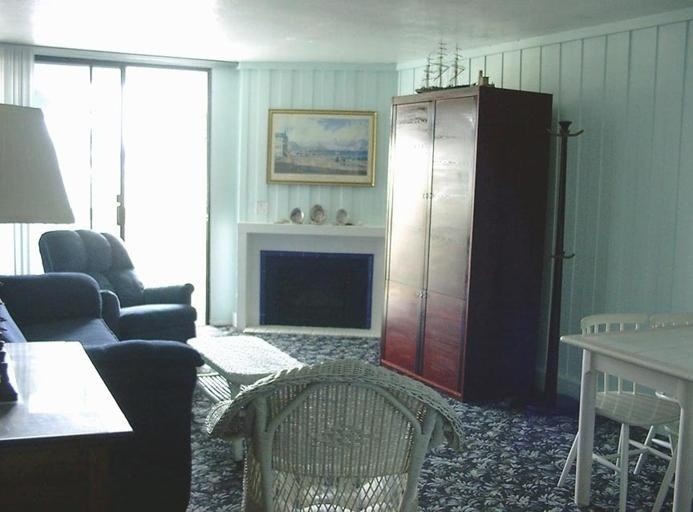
[
  {"xmin": 0, "ymin": 340, "xmax": 134, "ymax": 510},
  {"xmin": 560, "ymin": 327, "xmax": 693, "ymax": 512},
  {"xmin": 186, "ymin": 334, "xmax": 313, "ymax": 404}
]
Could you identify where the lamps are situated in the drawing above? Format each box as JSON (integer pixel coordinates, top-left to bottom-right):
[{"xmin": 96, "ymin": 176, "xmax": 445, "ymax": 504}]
[{"xmin": 1, "ymin": 103, "xmax": 77, "ymax": 404}]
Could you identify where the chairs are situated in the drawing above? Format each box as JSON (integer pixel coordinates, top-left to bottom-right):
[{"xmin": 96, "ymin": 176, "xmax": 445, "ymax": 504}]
[
  {"xmin": 204, "ymin": 356, "xmax": 466, "ymax": 512},
  {"xmin": 557, "ymin": 312, "xmax": 680, "ymax": 512},
  {"xmin": 38, "ymin": 229, "xmax": 198, "ymax": 343},
  {"xmin": 633, "ymin": 312, "xmax": 693, "ymax": 476}
]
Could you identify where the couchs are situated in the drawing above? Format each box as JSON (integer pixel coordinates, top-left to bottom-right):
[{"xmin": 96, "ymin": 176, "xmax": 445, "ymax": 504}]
[{"xmin": 1, "ymin": 271, "xmax": 204, "ymax": 511}]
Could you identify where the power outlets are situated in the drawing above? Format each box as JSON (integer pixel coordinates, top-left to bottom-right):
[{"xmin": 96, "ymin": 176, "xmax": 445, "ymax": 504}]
[{"xmin": 256, "ymin": 200, "xmax": 268, "ymax": 216}]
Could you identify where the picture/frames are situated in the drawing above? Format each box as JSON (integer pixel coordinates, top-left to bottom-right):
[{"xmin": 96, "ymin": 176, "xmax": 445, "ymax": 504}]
[
  {"xmin": 266, "ymin": 108, "xmax": 378, "ymax": 187},
  {"xmin": 381, "ymin": 85, "xmax": 554, "ymax": 402}
]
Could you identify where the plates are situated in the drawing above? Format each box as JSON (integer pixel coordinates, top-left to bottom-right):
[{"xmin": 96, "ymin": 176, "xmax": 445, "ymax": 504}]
[
  {"xmin": 335, "ymin": 208, "xmax": 348, "ymax": 225},
  {"xmin": 310, "ymin": 204, "xmax": 327, "ymax": 223},
  {"xmin": 290, "ymin": 208, "xmax": 304, "ymax": 224}
]
